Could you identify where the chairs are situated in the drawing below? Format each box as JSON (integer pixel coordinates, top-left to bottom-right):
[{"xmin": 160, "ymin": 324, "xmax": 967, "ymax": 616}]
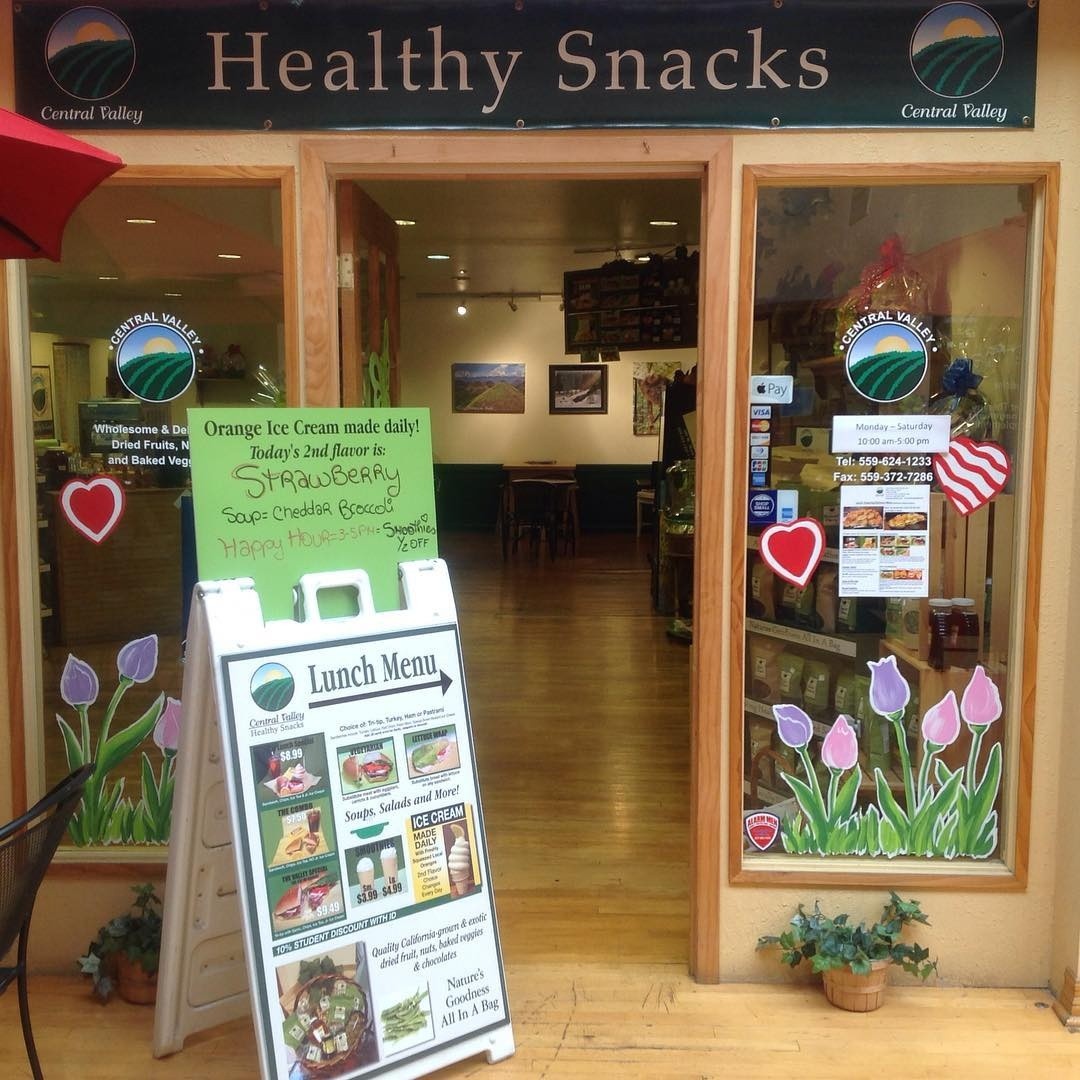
[
  {"xmin": 1, "ymin": 762, "xmax": 97, "ymax": 1080},
  {"xmin": 496, "ymin": 476, "xmax": 580, "ymax": 558}
]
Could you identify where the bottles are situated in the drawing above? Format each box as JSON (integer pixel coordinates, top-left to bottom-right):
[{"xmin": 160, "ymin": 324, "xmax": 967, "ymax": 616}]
[
  {"xmin": 928, "ymin": 597, "xmax": 981, "ymax": 672},
  {"xmin": 196, "ymin": 345, "xmax": 218, "ymax": 377},
  {"xmin": 43, "ymin": 446, "xmax": 152, "ymax": 491},
  {"xmin": 219, "ymin": 343, "xmax": 247, "ymax": 379}
]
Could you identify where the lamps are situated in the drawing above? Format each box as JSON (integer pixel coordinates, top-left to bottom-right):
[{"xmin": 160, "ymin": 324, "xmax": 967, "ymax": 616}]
[
  {"xmin": 457, "ymin": 299, "xmax": 468, "ymax": 315},
  {"xmin": 508, "ymin": 296, "xmax": 516, "ymax": 312}
]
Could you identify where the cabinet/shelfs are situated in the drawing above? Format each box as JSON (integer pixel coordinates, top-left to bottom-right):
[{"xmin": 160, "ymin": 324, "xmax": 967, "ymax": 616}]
[
  {"xmin": 36, "ymin": 473, "xmax": 53, "ymax": 619},
  {"xmin": 745, "ymin": 349, "xmax": 1010, "ymax": 835}
]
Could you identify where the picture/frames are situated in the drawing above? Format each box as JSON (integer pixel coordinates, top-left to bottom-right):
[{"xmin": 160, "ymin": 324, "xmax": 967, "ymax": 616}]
[
  {"xmin": 33, "ymin": 365, "xmax": 54, "ymax": 440},
  {"xmin": 548, "ymin": 363, "xmax": 608, "ymax": 415}
]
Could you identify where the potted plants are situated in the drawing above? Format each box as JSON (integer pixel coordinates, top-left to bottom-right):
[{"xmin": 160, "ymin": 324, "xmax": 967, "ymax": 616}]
[
  {"xmin": 79, "ymin": 879, "xmax": 163, "ymax": 1006},
  {"xmin": 757, "ymin": 889, "xmax": 939, "ymax": 1012}
]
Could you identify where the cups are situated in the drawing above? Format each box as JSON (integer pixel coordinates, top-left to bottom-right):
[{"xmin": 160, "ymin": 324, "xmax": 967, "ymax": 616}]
[
  {"xmin": 307, "ymin": 810, "xmax": 320, "ymax": 832},
  {"xmin": 381, "ymin": 854, "xmax": 398, "ymax": 886},
  {"xmin": 357, "ymin": 866, "xmax": 375, "ymax": 900},
  {"xmin": 269, "ymin": 754, "xmax": 281, "ymax": 778}
]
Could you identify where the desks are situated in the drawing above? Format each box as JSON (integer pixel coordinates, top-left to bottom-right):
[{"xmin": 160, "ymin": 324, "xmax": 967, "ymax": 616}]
[{"xmin": 499, "ymin": 459, "xmax": 580, "ymax": 555}]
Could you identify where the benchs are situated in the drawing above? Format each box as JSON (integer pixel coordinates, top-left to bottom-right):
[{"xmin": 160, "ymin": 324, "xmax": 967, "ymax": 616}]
[{"xmin": 637, "ymin": 480, "xmax": 657, "ymax": 536}]
